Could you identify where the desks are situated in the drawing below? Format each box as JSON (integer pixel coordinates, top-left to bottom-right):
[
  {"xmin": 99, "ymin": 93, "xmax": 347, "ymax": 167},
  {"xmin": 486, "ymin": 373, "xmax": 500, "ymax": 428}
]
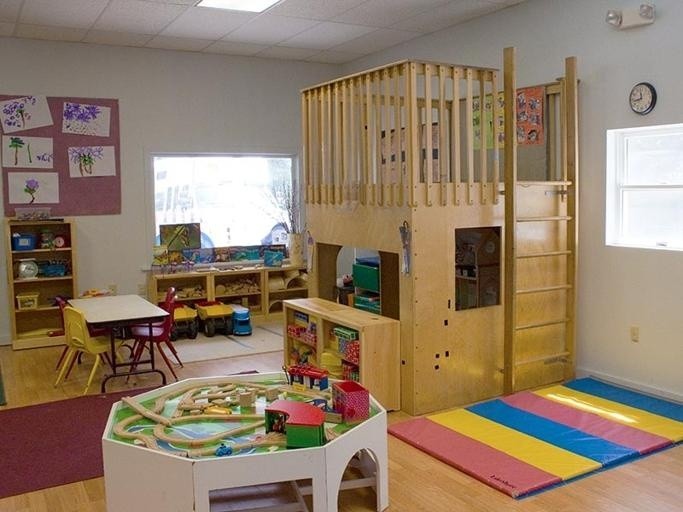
[{"xmin": 102, "ymin": 370, "xmax": 390, "ymax": 512}]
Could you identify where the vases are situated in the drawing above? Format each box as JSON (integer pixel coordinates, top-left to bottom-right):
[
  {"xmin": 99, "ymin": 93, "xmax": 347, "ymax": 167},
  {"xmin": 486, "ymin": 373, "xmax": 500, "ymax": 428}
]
[{"xmin": 287, "ymin": 232, "xmax": 304, "ymax": 265}]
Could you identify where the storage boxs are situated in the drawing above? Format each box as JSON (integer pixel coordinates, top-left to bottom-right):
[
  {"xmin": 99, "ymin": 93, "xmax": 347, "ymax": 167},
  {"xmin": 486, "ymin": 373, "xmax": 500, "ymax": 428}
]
[
  {"xmin": 351, "ymin": 263, "xmax": 379, "ymax": 292},
  {"xmin": 353, "ymin": 294, "xmax": 380, "ymax": 314}
]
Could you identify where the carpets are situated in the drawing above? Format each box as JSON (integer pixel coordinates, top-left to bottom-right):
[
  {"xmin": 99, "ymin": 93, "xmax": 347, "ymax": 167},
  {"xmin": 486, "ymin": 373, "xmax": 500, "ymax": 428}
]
[{"xmin": 0, "ymin": 371, "xmax": 260, "ymax": 499}]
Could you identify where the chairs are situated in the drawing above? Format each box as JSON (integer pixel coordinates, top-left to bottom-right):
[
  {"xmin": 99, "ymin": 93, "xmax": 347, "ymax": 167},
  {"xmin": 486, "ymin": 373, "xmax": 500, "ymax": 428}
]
[{"xmin": 55, "ymin": 286, "xmax": 178, "ymax": 396}]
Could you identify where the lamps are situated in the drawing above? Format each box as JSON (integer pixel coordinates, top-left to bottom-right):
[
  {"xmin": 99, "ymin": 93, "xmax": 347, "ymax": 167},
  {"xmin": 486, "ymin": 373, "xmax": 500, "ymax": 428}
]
[{"xmin": 605, "ymin": 2, "xmax": 655, "ymax": 30}]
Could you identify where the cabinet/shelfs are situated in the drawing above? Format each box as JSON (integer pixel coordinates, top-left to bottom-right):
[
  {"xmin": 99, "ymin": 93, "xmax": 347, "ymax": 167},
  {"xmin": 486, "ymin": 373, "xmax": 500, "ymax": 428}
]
[
  {"xmin": 282, "ymin": 298, "xmax": 402, "ymax": 416},
  {"xmin": 455, "ymin": 228, "xmax": 500, "ymax": 311},
  {"xmin": 4, "ymin": 221, "xmax": 79, "ymax": 349},
  {"xmin": 352, "ymin": 247, "xmax": 400, "ymax": 319},
  {"xmin": 144, "ymin": 265, "xmax": 308, "ymax": 315}
]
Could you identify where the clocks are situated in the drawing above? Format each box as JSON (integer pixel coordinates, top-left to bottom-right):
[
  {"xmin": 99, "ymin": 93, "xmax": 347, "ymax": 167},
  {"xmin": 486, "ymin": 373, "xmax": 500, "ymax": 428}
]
[{"xmin": 629, "ymin": 82, "xmax": 657, "ymax": 114}]
